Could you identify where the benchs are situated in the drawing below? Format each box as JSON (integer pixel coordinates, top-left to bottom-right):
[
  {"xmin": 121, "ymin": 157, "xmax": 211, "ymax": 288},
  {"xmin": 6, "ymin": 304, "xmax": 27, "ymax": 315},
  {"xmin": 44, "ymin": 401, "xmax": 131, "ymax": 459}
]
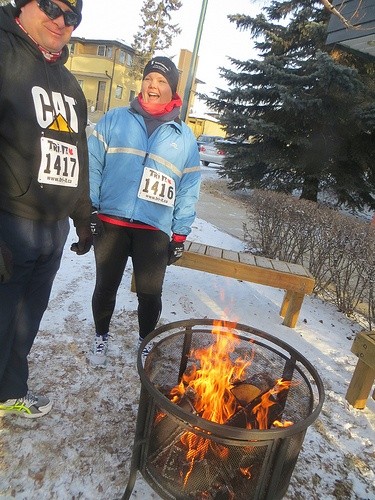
[
  {"xmin": 345, "ymin": 331, "xmax": 375, "ymax": 408},
  {"xmin": 130, "ymin": 241, "xmax": 315, "ymax": 328}
]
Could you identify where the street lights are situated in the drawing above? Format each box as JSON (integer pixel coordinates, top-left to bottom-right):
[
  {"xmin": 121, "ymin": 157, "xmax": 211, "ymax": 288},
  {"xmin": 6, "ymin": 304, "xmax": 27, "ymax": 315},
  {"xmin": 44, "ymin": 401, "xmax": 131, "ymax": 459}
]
[{"xmin": 105, "ymin": 44, "xmax": 122, "ymax": 111}]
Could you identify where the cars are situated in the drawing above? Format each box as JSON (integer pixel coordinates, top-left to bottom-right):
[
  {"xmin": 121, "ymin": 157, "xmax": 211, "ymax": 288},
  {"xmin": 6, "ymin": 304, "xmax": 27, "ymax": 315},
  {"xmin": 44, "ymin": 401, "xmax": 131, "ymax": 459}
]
[{"xmin": 196, "ymin": 135, "xmax": 249, "ymax": 168}]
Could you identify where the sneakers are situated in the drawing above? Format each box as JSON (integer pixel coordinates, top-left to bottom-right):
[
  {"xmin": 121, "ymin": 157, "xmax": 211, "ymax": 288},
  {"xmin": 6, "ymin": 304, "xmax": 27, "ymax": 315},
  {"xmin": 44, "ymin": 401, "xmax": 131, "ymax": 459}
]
[
  {"xmin": 0, "ymin": 392, "xmax": 52, "ymax": 419},
  {"xmin": 88, "ymin": 334, "xmax": 109, "ymax": 369},
  {"xmin": 137, "ymin": 336, "xmax": 151, "ymax": 368}
]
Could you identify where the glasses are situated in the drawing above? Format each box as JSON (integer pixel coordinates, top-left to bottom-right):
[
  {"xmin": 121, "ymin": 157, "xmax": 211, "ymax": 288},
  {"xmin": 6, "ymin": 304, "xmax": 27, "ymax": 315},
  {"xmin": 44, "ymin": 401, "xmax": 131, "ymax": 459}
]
[{"xmin": 35, "ymin": 0, "xmax": 79, "ymax": 26}]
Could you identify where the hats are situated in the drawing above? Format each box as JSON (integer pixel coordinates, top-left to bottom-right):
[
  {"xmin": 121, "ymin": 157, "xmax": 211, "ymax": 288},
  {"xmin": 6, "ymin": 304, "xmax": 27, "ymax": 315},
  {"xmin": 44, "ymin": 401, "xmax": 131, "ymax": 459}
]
[
  {"xmin": 60, "ymin": 0, "xmax": 82, "ymax": 31},
  {"xmin": 143, "ymin": 57, "xmax": 178, "ymax": 98}
]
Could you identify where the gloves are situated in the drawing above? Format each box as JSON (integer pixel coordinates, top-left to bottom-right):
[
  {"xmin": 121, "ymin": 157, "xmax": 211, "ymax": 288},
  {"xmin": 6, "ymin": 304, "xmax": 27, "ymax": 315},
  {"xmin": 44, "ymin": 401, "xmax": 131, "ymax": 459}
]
[
  {"xmin": 167, "ymin": 233, "xmax": 188, "ymax": 265},
  {"xmin": 91, "ymin": 207, "xmax": 105, "ymax": 235},
  {"xmin": 70, "ymin": 227, "xmax": 93, "ymax": 255}
]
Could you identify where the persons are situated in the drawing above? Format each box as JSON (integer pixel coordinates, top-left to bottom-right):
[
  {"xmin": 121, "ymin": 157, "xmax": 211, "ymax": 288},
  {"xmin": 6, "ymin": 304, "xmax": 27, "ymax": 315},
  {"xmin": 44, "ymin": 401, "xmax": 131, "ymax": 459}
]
[
  {"xmin": 80, "ymin": 56, "xmax": 201, "ymax": 369},
  {"xmin": 0, "ymin": 0, "xmax": 93, "ymax": 418}
]
